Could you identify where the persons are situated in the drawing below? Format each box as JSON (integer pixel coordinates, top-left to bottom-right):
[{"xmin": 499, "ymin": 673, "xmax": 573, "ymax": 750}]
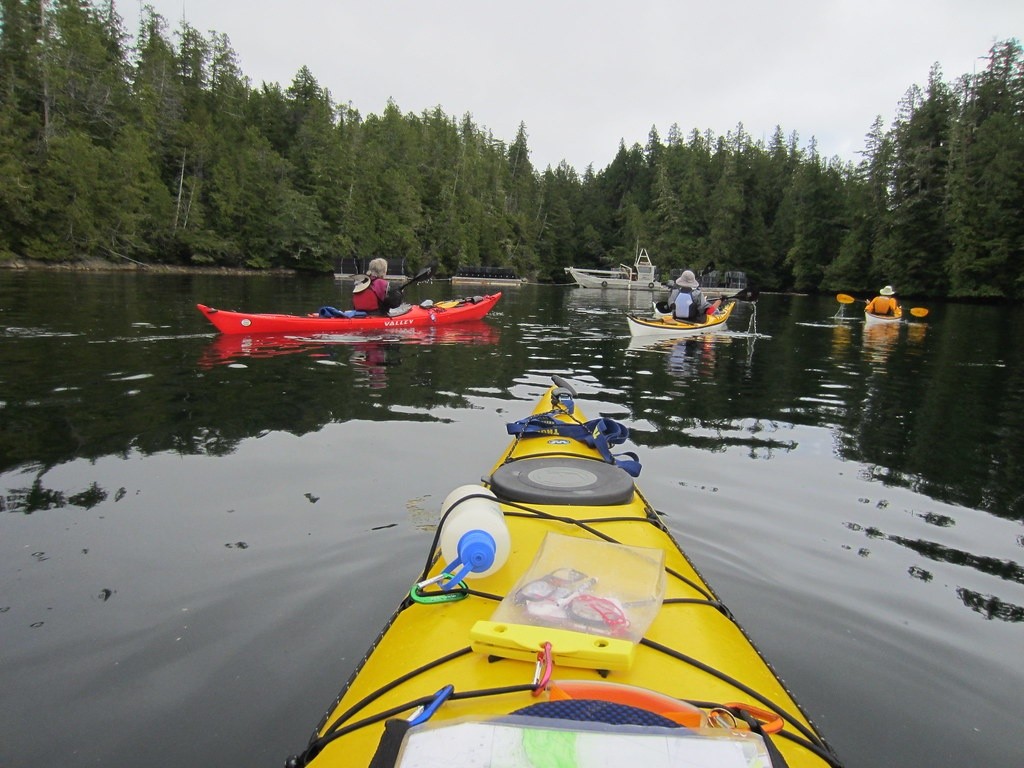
[
  {"xmin": 352, "ymin": 258, "xmax": 391, "ymax": 317},
  {"xmin": 667, "ymin": 270, "xmax": 713, "ymax": 319},
  {"xmin": 865, "ymin": 286, "xmax": 902, "ymax": 318}
]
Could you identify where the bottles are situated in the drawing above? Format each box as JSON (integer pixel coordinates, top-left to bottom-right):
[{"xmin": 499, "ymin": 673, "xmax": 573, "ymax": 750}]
[{"xmin": 438, "ymin": 484, "xmax": 510, "ymax": 592}]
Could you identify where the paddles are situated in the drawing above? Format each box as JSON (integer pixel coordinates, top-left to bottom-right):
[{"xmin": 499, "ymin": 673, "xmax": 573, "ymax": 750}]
[
  {"xmin": 706, "ymin": 286, "xmax": 759, "ymax": 302},
  {"xmin": 837, "ymin": 293, "xmax": 928, "ymax": 317},
  {"xmin": 396, "ymin": 267, "xmax": 432, "ymax": 292}
]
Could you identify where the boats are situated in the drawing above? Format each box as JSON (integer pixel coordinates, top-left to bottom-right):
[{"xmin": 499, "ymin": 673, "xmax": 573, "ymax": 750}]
[
  {"xmin": 563, "ymin": 247, "xmax": 749, "ymax": 295},
  {"xmin": 195, "ymin": 290, "xmax": 504, "ymax": 336},
  {"xmin": 295, "ymin": 380, "xmax": 842, "ymax": 768},
  {"xmin": 862, "ymin": 310, "xmax": 904, "ymax": 324},
  {"xmin": 626, "ymin": 301, "xmax": 736, "ymax": 337}
]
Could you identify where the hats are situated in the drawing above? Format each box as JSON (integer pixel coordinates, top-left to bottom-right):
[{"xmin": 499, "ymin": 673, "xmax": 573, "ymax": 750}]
[
  {"xmin": 675, "ymin": 270, "xmax": 700, "ymax": 289},
  {"xmin": 879, "ymin": 285, "xmax": 896, "ymax": 295},
  {"xmin": 348, "ymin": 272, "xmax": 372, "ymax": 293}
]
